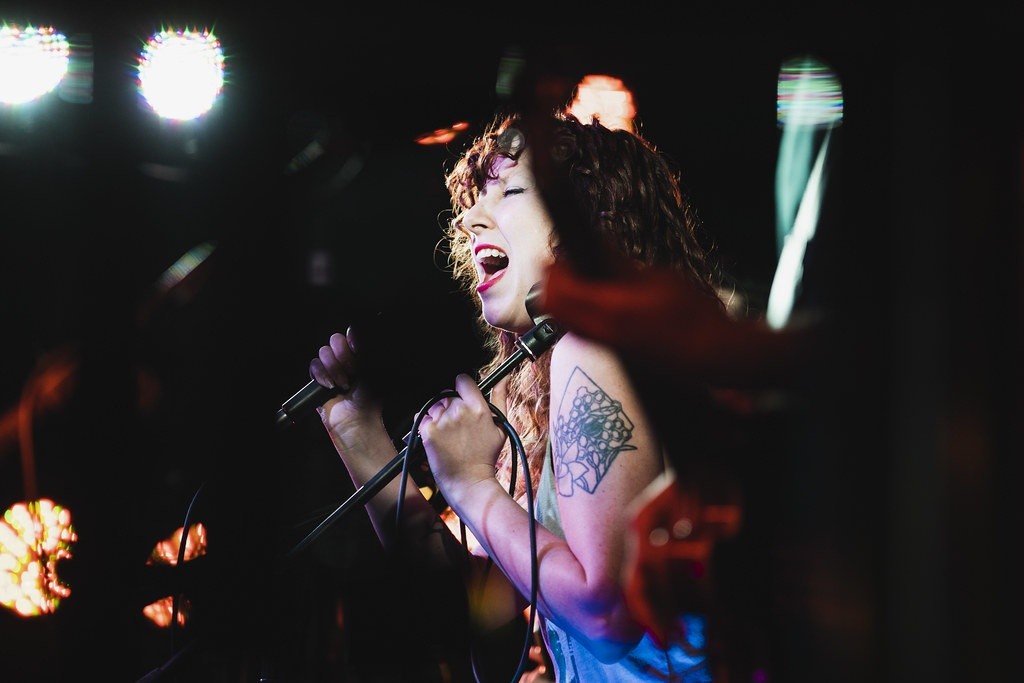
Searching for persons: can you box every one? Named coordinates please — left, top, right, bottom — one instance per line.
left=309, top=0, right=1024, bottom=683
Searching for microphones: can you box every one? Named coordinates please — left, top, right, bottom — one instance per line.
left=271, top=305, right=401, bottom=434
